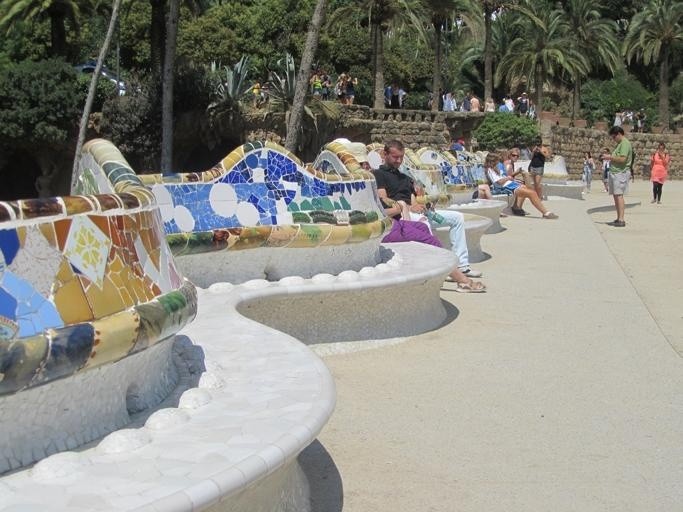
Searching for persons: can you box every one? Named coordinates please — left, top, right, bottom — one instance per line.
left=254, top=77, right=266, bottom=98
left=614, top=108, right=646, bottom=133
left=384, top=81, right=407, bottom=109
left=650, top=143, right=669, bottom=204
left=439, top=88, right=537, bottom=119
left=440, top=136, right=558, bottom=219
left=309, top=71, right=359, bottom=105
left=35, top=159, right=59, bottom=197
left=582, top=151, right=595, bottom=194
left=364, top=140, right=485, bottom=292
left=601, top=148, right=611, bottom=192
left=601, top=126, right=633, bottom=227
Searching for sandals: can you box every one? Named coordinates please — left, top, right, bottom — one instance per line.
left=456, top=279, right=486, bottom=292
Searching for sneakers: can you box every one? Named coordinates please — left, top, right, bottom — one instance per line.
left=512, top=207, right=524, bottom=216
left=608, top=220, right=625, bottom=226
left=543, top=211, right=557, bottom=218
left=458, top=266, right=481, bottom=277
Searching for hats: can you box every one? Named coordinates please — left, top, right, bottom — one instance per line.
left=521, top=92, right=529, bottom=97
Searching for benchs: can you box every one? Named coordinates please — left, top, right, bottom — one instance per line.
left=0, top=178, right=585, bottom=512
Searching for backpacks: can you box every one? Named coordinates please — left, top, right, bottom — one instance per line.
left=520, top=100, right=527, bottom=113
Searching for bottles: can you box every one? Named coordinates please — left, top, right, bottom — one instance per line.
left=426, top=211, right=445, bottom=225
left=581, top=191, right=585, bottom=200
